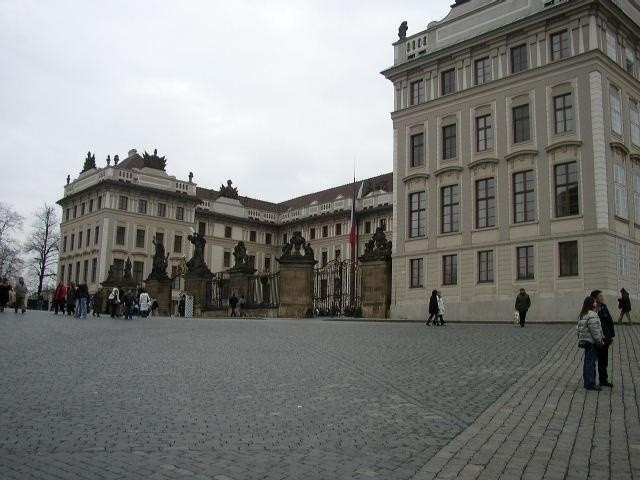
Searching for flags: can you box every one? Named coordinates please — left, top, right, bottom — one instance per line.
left=348, top=204, right=356, bottom=246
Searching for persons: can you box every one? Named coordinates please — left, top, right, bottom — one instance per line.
left=432, top=289, right=446, bottom=326
left=616, top=288, right=632, bottom=326
left=50, top=280, right=160, bottom=321
left=590, top=289, right=615, bottom=388
left=221, top=292, right=247, bottom=318
left=576, top=296, right=605, bottom=391
left=425, top=290, right=441, bottom=326
left=0, top=276, right=29, bottom=315
left=515, top=288, right=531, bottom=327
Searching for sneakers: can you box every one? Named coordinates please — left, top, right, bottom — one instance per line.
left=521, top=324, right=524, bottom=327
left=584, top=385, right=602, bottom=391
left=426, top=323, right=446, bottom=326
left=15, top=310, right=26, bottom=314
left=54, top=311, right=147, bottom=320
left=599, top=381, right=613, bottom=387
